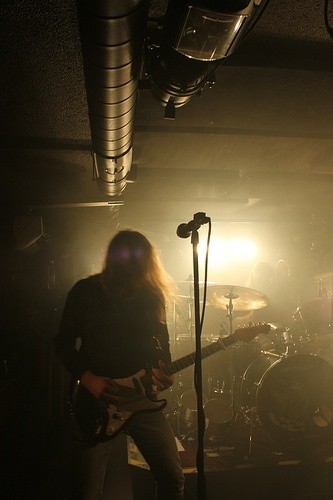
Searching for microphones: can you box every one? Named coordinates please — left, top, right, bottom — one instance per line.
left=177, top=217, right=210, bottom=238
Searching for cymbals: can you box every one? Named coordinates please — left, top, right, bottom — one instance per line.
left=195, top=283, right=271, bottom=309
left=171, top=280, right=207, bottom=299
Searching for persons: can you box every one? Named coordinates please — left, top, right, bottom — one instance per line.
left=252, top=260, right=296, bottom=306
left=55, top=229, right=186, bottom=500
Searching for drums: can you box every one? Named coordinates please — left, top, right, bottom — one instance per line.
left=169, top=332, right=207, bottom=363
left=209, top=333, right=265, bottom=396
left=240, top=349, right=333, bottom=440
left=200, top=373, right=234, bottom=423
left=179, top=368, right=209, bottom=410
left=288, top=299, right=333, bottom=346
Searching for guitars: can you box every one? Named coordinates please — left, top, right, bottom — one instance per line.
left=75, top=321, right=272, bottom=449
